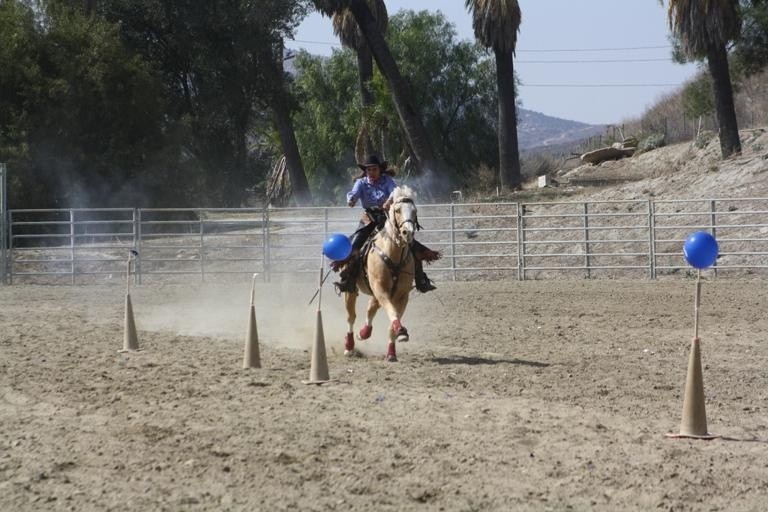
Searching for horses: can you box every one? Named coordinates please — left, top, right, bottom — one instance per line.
left=343, top=184, right=418, bottom=362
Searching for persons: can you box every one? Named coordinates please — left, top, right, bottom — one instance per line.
left=331, top=156, right=438, bottom=292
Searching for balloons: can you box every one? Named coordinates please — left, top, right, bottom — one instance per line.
left=682, top=231, right=719, bottom=270
left=323, top=233, right=352, bottom=262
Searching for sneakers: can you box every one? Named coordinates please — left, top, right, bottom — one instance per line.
left=333, top=279, right=355, bottom=293
left=417, top=284, right=436, bottom=293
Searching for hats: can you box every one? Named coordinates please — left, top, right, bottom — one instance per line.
left=356, top=155, right=388, bottom=175
